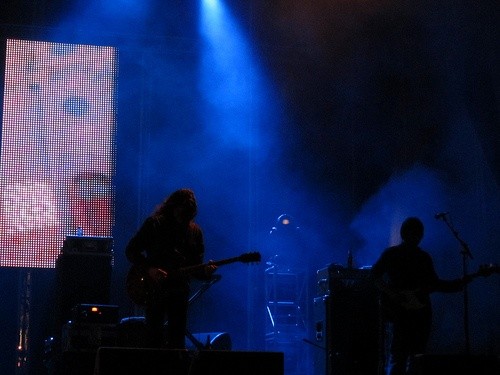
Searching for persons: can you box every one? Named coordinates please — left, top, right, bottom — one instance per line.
left=126, top=189, right=218, bottom=349
left=368, top=217, right=472, bottom=375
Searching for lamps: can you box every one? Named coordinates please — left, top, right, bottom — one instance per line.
left=270, top=213, right=305, bottom=262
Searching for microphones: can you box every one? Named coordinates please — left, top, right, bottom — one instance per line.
left=435, top=211, right=448, bottom=219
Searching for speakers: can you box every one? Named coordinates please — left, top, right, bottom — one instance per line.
left=312, top=295, right=384, bottom=375
left=63, top=235, right=112, bottom=323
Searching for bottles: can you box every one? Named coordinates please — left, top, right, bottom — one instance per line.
left=77, top=227, right=82, bottom=236
left=346, top=250, right=352, bottom=267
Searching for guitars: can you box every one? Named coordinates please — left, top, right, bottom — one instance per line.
left=126, top=251, right=261, bottom=299
left=382, top=264, right=500, bottom=323
